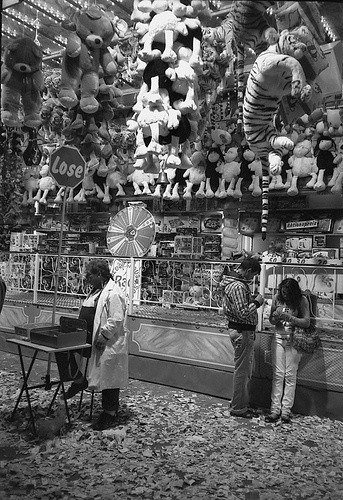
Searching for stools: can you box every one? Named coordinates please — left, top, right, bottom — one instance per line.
left=76, top=351, right=127, bottom=419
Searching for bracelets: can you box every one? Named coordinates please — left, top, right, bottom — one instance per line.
left=289, top=315, right=295, bottom=323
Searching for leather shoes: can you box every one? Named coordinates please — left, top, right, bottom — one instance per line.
left=94, top=411, right=119, bottom=430
left=61, top=377, right=88, bottom=399
left=230, top=409, right=260, bottom=418
left=247, top=402, right=257, bottom=408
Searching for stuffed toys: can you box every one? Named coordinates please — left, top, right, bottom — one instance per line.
left=0, top=0, right=343, bottom=208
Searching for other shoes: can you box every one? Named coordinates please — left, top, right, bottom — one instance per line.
left=280, top=413, right=290, bottom=424
left=265, top=412, right=279, bottom=424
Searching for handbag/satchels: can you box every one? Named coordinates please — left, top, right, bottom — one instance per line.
left=293, top=294, right=321, bottom=353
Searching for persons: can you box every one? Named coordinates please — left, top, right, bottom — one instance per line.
left=220, top=258, right=263, bottom=419
left=266, top=278, right=310, bottom=423
left=55, top=259, right=129, bottom=431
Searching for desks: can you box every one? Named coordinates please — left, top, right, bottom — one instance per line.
left=5, top=339, right=91, bottom=436
